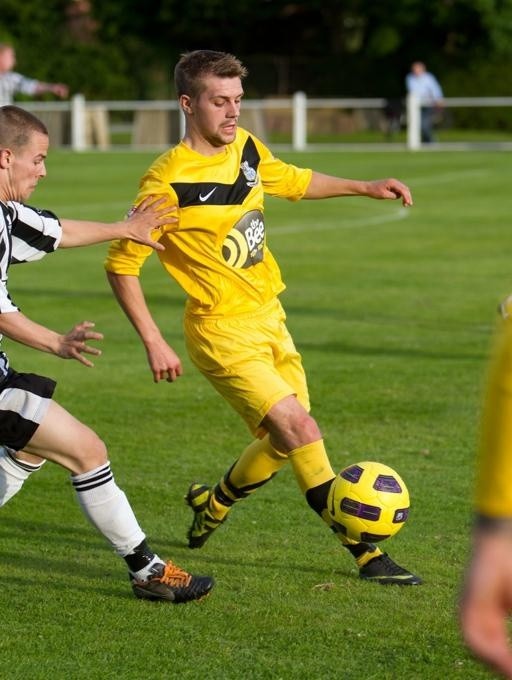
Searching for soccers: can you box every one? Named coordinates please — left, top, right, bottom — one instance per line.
left=326, top=460, right=409, bottom=543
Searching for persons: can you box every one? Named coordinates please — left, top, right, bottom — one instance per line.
left=0, top=103, right=215, bottom=603
left=0, top=40, right=69, bottom=107
left=104, top=48, right=426, bottom=587
left=458, top=293, right=512, bottom=679
left=406, top=60, right=445, bottom=141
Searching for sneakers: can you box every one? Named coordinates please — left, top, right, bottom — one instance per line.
left=127, top=553, right=216, bottom=604
left=183, top=482, right=228, bottom=549
left=358, top=553, right=422, bottom=585
